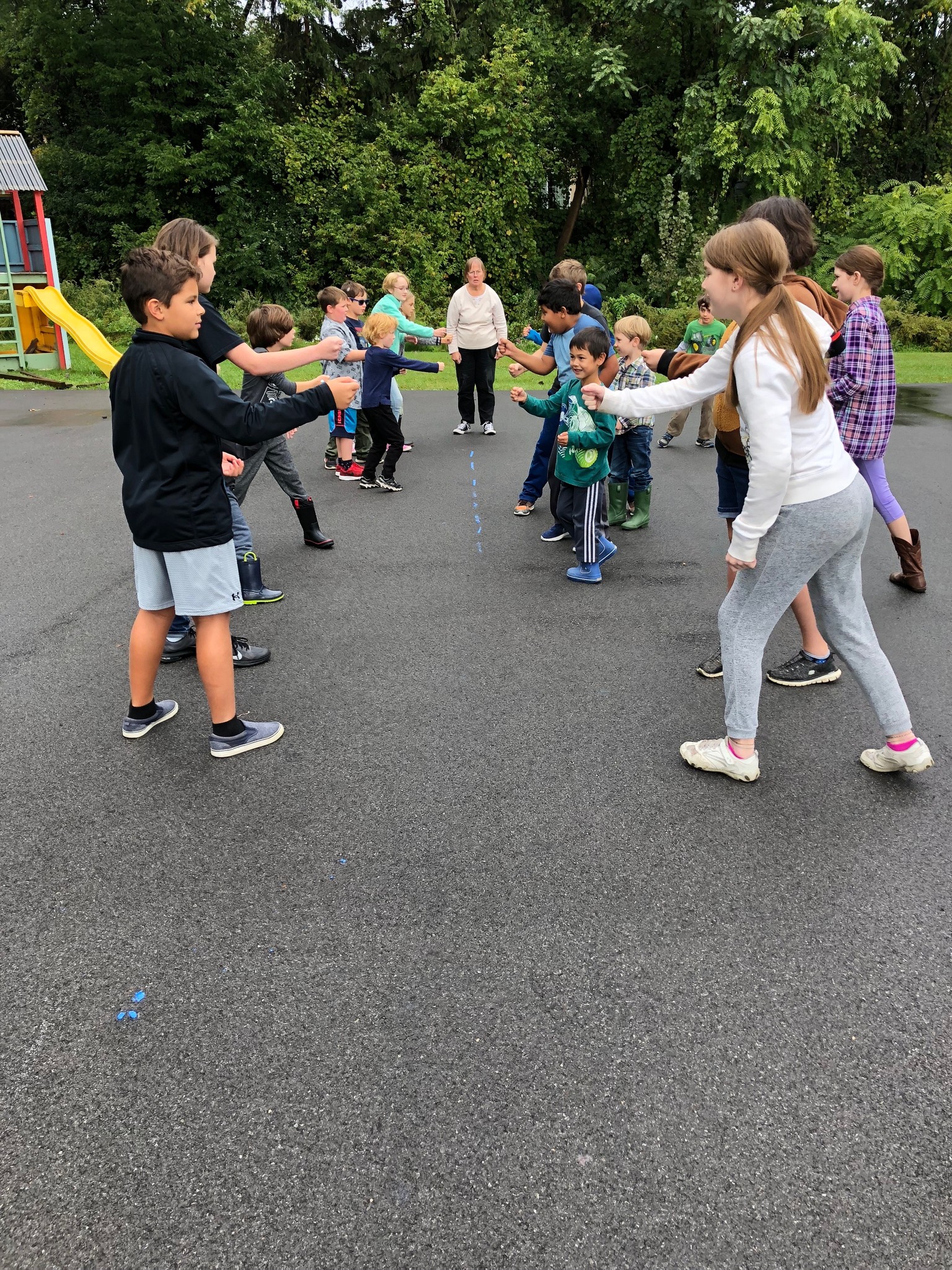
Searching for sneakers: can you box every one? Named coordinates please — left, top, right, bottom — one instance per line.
left=657, top=431, right=674, bottom=448
left=231, top=634, right=271, bottom=668
left=159, top=625, right=197, bottom=664
left=540, top=523, right=569, bottom=541
left=696, top=645, right=723, bottom=678
left=359, top=476, right=379, bottom=489
left=453, top=420, right=471, bottom=434
left=565, top=535, right=617, bottom=583
left=122, top=700, right=179, bottom=739
left=860, top=738, right=935, bottom=774
left=355, top=456, right=385, bottom=464
left=766, top=650, right=841, bottom=687
left=375, top=475, right=403, bottom=492
left=513, top=498, right=535, bottom=516
left=482, top=421, right=496, bottom=435
left=680, top=735, right=760, bottom=783
left=324, top=457, right=337, bottom=469
left=386, top=444, right=413, bottom=452
left=696, top=437, right=715, bottom=448
left=209, top=718, right=285, bottom=758
left=335, top=463, right=362, bottom=481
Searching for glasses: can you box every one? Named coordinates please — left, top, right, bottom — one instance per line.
left=349, top=296, right=370, bottom=306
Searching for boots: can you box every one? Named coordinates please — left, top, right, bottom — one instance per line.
left=292, top=497, right=335, bottom=548
left=889, top=527, right=927, bottom=593
left=237, top=552, right=285, bottom=606
left=602, top=481, right=652, bottom=530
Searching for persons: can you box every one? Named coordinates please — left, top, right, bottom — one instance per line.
left=821, top=245, right=927, bottom=592
left=639, top=197, right=841, bottom=689
left=578, top=217, right=935, bottom=783
left=658, top=295, right=727, bottom=448
left=227, top=302, right=335, bottom=549
left=109, top=217, right=362, bottom=759
left=447, top=256, right=507, bottom=436
left=317, top=272, right=454, bottom=490
left=498, top=258, right=658, bottom=584
